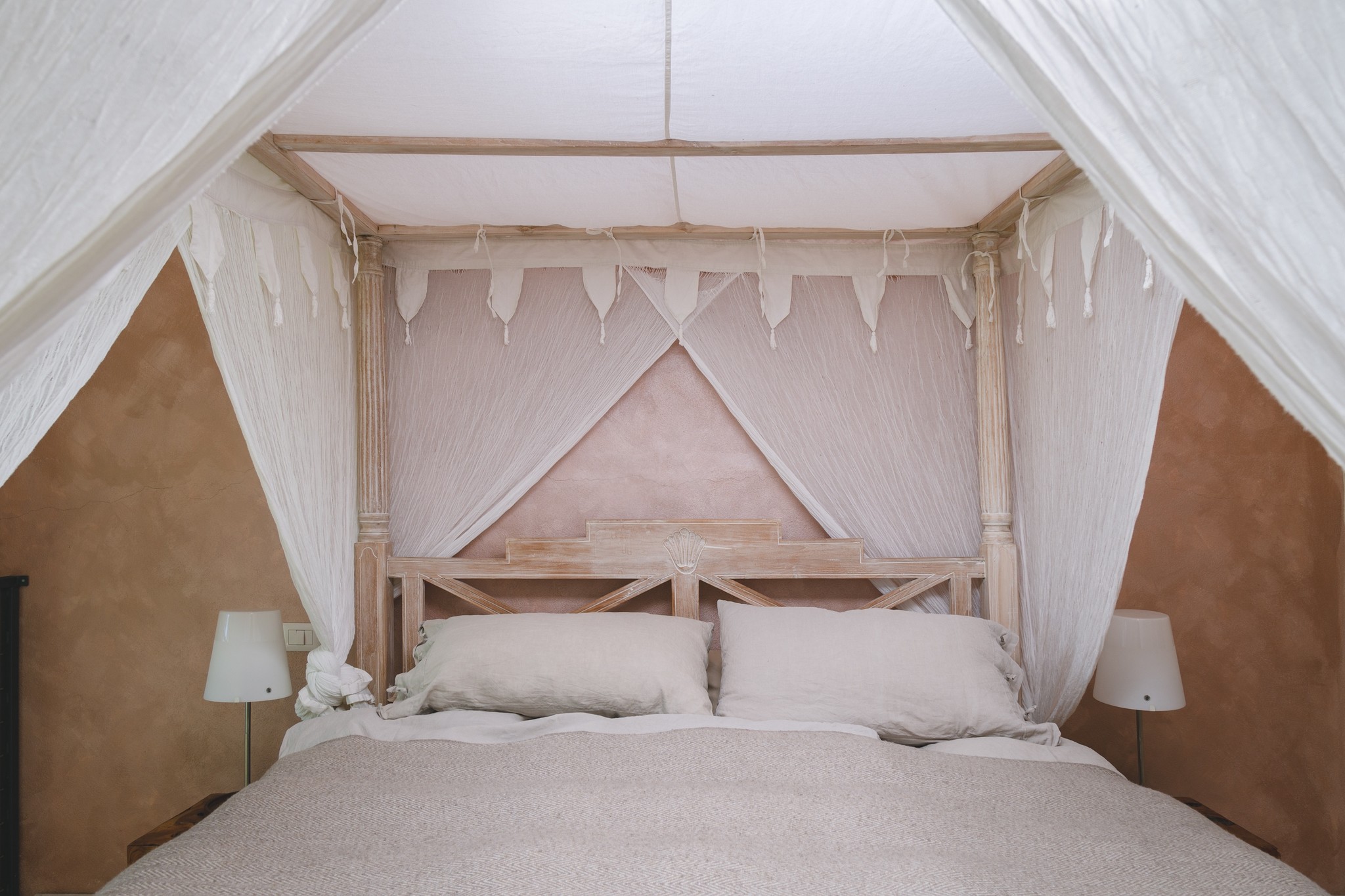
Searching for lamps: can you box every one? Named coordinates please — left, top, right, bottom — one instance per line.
left=1091, top=608, right=1188, bottom=786
left=201, top=610, right=294, bottom=786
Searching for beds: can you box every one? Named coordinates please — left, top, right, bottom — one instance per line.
left=84, top=515, right=1332, bottom=896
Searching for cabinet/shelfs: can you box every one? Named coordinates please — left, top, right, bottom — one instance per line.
left=0, top=574, right=30, bottom=896
left=125, top=792, right=229, bottom=869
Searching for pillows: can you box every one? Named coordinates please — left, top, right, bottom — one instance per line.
left=715, top=597, right=1064, bottom=747
left=374, top=611, right=715, bottom=719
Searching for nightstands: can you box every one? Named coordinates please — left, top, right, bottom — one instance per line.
left=1173, top=796, right=1281, bottom=857
